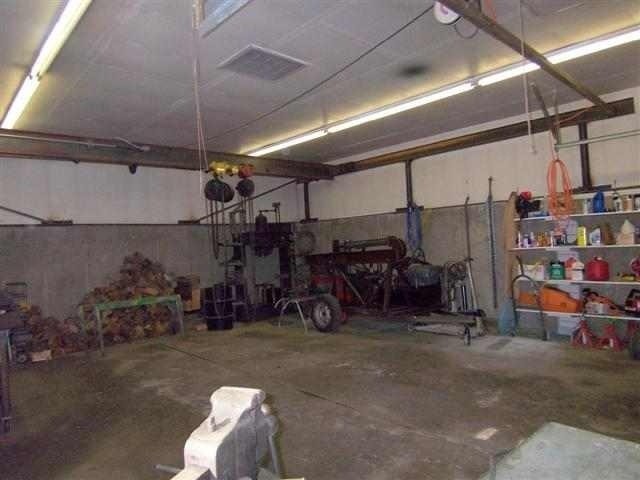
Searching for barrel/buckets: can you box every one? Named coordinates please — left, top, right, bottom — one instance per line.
left=204, top=286, right=235, bottom=331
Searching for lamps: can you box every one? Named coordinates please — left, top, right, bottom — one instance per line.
left=1, top=1, right=94, bottom=131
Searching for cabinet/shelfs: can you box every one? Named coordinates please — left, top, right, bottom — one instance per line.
left=497, top=191, right=640, bottom=336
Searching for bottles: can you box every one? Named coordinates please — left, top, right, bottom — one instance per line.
left=522, top=234, right=528, bottom=249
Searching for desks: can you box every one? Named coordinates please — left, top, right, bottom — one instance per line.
left=76, top=294, right=186, bottom=356
